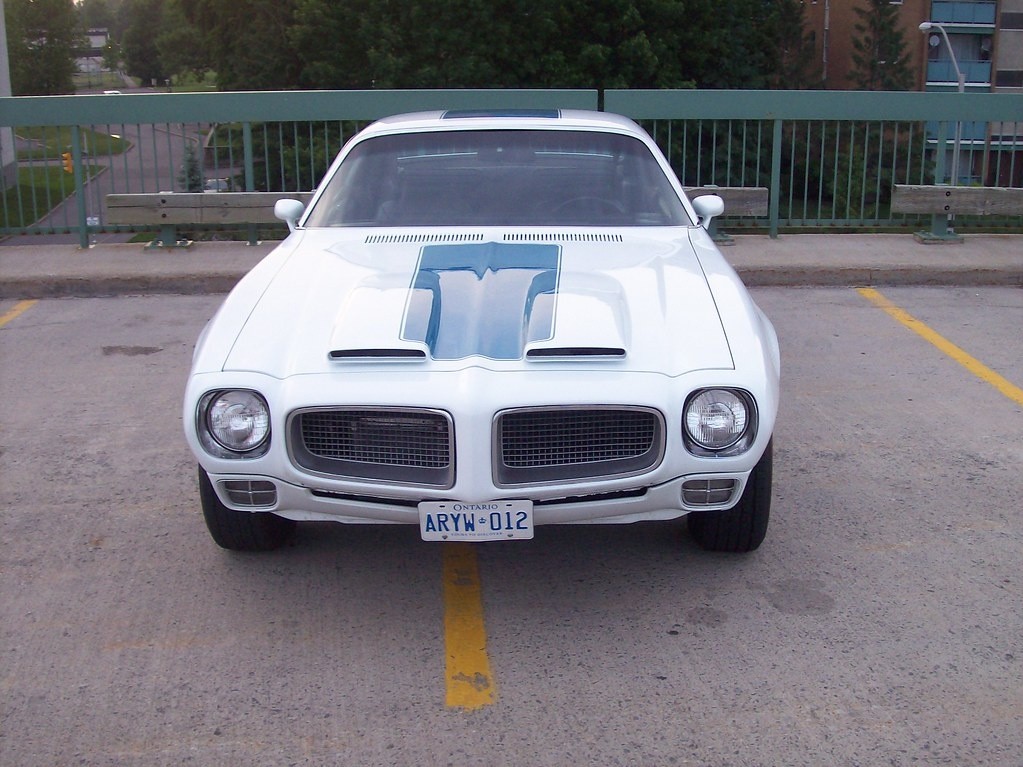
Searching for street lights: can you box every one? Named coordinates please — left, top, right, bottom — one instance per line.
left=920, top=21, right=965, bottom=228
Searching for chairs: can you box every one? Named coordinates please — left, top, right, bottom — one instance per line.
left=391, top=169, right=487, bottom=226
left=494, top=157, right=625, bottom=227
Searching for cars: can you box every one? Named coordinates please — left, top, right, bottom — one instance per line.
left=182, top=107, right=780, bottom=553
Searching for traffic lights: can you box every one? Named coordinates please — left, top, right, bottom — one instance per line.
left=63, top=151, right=72, bottom=173
